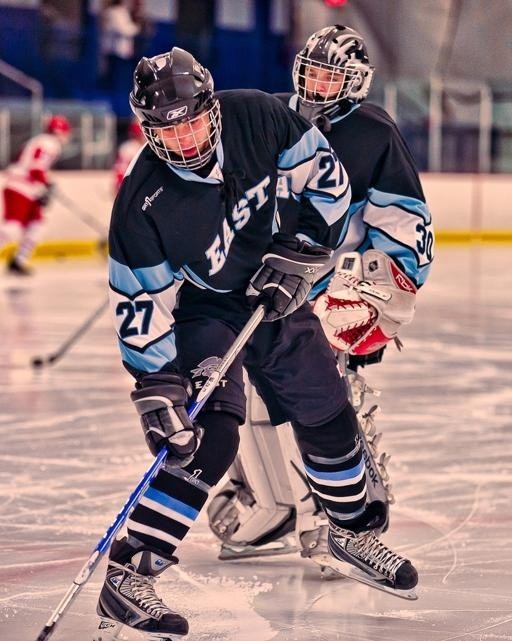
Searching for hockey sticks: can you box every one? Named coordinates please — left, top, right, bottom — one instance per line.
left=33, top=299, right=109, bottom=367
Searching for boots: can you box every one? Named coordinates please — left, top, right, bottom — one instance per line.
left=208, top=477, right=418, bottom=595
left=95, top=531, right=196, bottom=635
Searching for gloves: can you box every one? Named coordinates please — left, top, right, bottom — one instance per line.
left=129, top=366, right=202, bottom=470
left=245, top=232, right=332, bottom=323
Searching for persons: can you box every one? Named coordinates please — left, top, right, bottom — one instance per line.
left=96, top=0, right=143, bottom=122
left=1, top=115, right=72, bottom=278
left=95, top=45, right=419, bottom=638
left=113, top=117, right=149, bottom=196
left=205, top=24, right=436, bottom=560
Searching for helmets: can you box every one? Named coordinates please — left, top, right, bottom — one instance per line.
left=128, top=46, right=222, bottom=171
left=44, top=114, right=72, bottom=135
left=290, top=21, right=375, bottom=104
